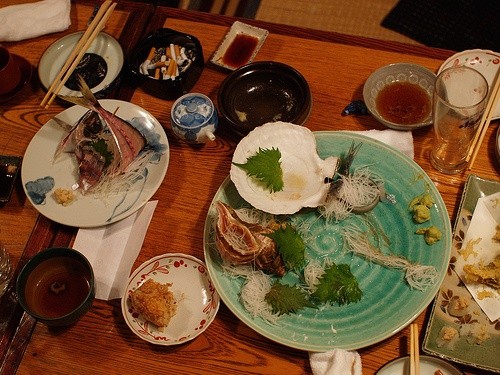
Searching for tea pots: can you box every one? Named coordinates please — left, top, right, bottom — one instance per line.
left=170, top=93, right=219, bottom=145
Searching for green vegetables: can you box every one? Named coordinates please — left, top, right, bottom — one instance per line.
left=232, top=146, right=284, bottom=193
left=91, top=136, right=115, bottom=169
left=261, top=222, right=363, bottom=315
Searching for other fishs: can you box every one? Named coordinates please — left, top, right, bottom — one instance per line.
left=52, top=75, right=150, bottom=194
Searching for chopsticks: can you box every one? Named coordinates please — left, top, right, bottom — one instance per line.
left=39, top=0, right=119, bottom=112
left=410, top=323, right=420, bottom=375
left=464, top=71, right=500, bottom=172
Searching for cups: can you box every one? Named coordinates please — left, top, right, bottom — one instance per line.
left=428, top=65, right=489, bottom=175
left=0, top=44, right=23, bottom=94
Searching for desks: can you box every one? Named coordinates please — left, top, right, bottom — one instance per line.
left=0, top=0, right=500, bottom=375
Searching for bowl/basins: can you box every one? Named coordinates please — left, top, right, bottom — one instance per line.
left=16, top=246, right=95, bottom=327
left=363, top=62, right=447, bottom=131
left=36, top=29, right=125, bottom=102
left=216, top=61, right=314, bottom=139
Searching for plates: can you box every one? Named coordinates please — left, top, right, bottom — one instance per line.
left=202, top=130, right=453, bottom=352
left=437, top=49, right=500, bottom=122
left=373, top=354, right=466, bottom=375
left=0, top=54, right=32, bottom=101
left=120, top=253, right=221, bottom=346
left=0, top=154, right=23, bottom=210
left=421, top=175, right=500, bottom=375
left=20, top=98, right=170, bottom=228
left=210, top=19, right=269, bottom=72
